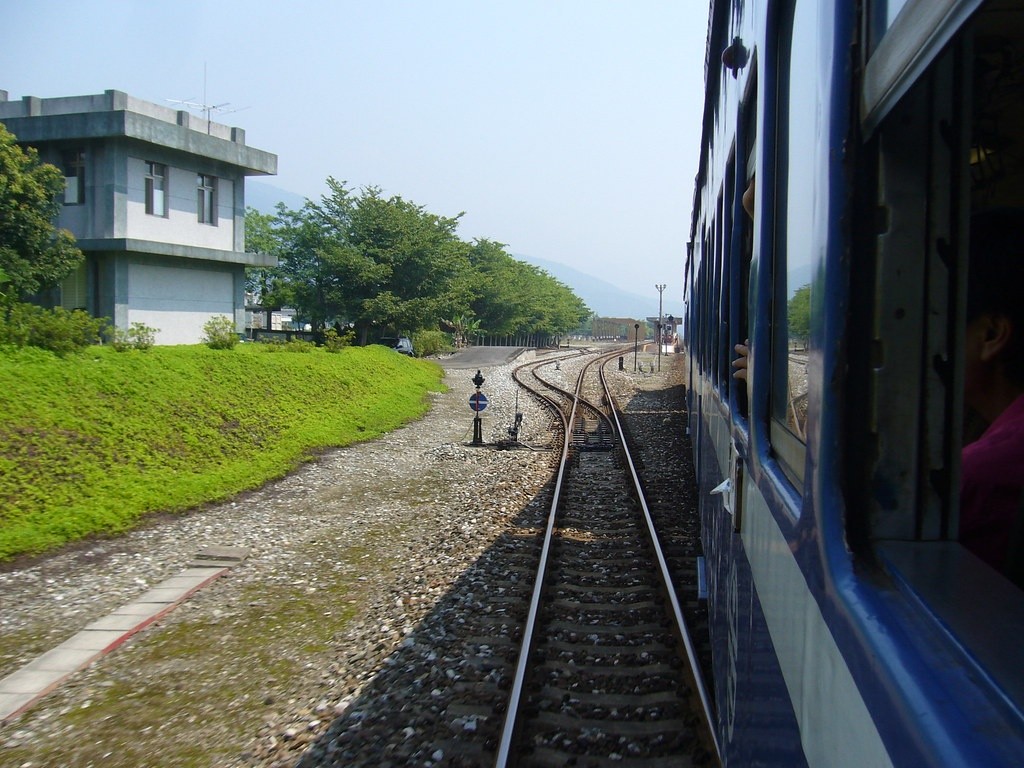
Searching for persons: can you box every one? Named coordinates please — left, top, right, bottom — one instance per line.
left=732, top=180, right=754, bottom=383
left=958, top=206, right=1024, bottom=574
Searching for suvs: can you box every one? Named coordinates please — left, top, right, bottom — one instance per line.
left=377, top=337, right=413, bottom=357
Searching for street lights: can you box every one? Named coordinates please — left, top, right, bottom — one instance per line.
left=654, top=284, right=667, bottom=353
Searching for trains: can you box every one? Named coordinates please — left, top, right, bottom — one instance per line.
left=685, top=0, right=1022, bottom=766
